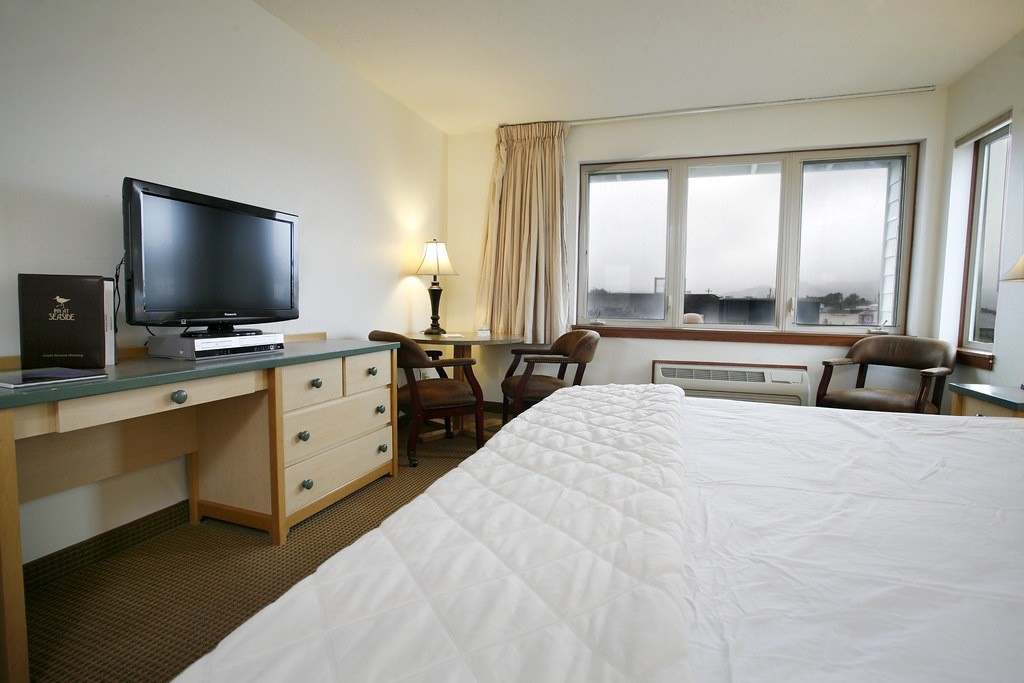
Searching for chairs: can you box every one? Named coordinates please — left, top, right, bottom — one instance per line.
left=501, top=329, right=601, bottom=427
left=368, top=329, right=485, bottom=469
left=815, top=335, right=957, bottom=416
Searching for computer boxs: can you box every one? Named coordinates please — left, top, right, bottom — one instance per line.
left=18, top=273, right=116, bottom=368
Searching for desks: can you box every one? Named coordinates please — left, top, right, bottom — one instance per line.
left=399, top=331, right=525, bottom=442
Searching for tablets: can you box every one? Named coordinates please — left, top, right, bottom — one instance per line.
left=0, top=366, right=108, bottom=389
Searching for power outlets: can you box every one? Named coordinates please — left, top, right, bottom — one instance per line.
left=420, top=369, right=430, bottom=381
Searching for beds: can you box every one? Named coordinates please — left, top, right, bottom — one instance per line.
left=165, top=382, right=1024, bottom=683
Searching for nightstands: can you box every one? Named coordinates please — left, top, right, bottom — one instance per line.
left=948, top=382, right=1024, bottom=419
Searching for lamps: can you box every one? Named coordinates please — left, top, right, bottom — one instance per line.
left=414, top=238, right=459, bottom=336
left=998, top=253, right=1024, bottom=389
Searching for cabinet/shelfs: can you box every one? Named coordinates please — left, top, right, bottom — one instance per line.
left=0, top=331, right=400, bottom=683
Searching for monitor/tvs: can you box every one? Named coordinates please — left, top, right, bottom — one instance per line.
left=122, top=176, right=300, bottom=326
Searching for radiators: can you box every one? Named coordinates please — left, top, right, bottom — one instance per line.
left=651, top=360, right=810, bottom=407
